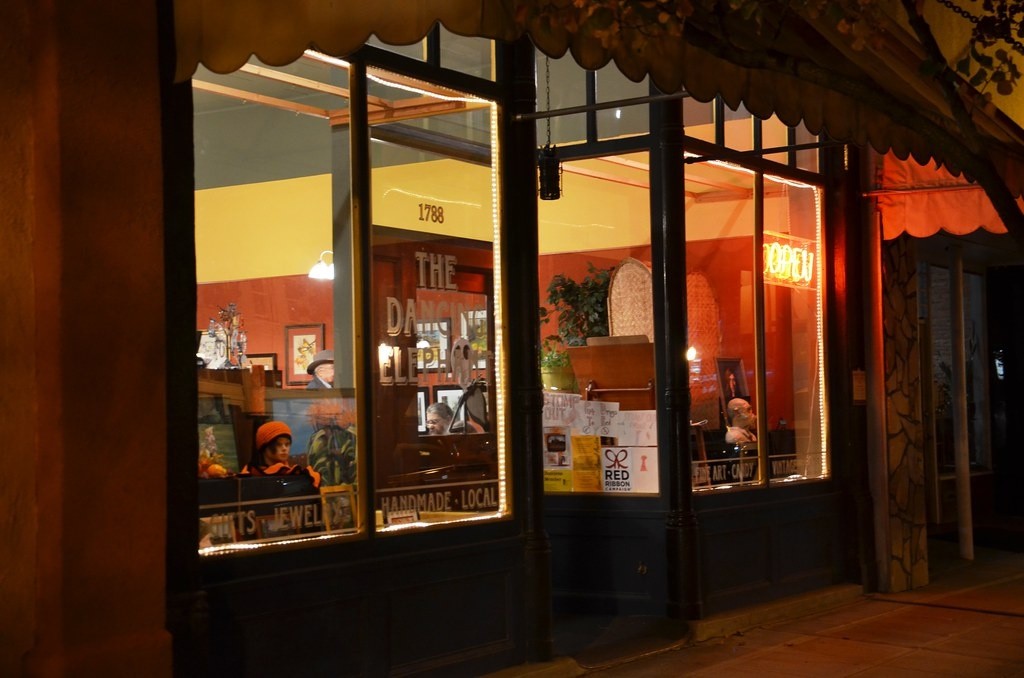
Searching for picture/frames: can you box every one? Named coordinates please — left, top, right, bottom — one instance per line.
left=714, top=357, right=752, bottom=430
left=285, top=323, right=326, bottom=386
left=417, top=387, right=430, bottom=434
left=239, top=353, right=277, bottom=371
left=320, top=484, right=357, bottom=535
left=415, top=317, right=452, bottom=373
left=263, top=388, right=358, bottom=530
left=459, top=308, right=487, bottom=370
left=433, top=385, right=489, bottom=433
left=197, top=329, right=231, bottom=368
left=382, top=505, right=421, bottom=524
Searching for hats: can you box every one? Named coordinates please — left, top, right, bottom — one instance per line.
left=256, top=422, right=291, bottom=451
left=307, top=350, right=334, bottom=375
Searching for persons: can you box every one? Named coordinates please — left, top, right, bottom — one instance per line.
left=724, top=367, right=737, bottom=399
left=425, top=402, right=476, bottom=434
left=307, top=351, right=335, bottom=390
left=725, top=399, right=757, bottom=443
left=240, top=421, right=322, bottom=490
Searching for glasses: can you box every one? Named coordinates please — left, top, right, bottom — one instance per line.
left=426, top=419, right=442, bottom=424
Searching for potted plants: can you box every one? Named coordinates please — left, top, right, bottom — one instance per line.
left=937, top=318, right=977, bottom=465
left=541, top=340, right=575, bottom=391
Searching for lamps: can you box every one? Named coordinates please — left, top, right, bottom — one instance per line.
left=308, top=251, right=336, bottom=280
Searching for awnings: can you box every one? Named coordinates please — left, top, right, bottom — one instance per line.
left=172, top=1, right=1024, bottom=244
